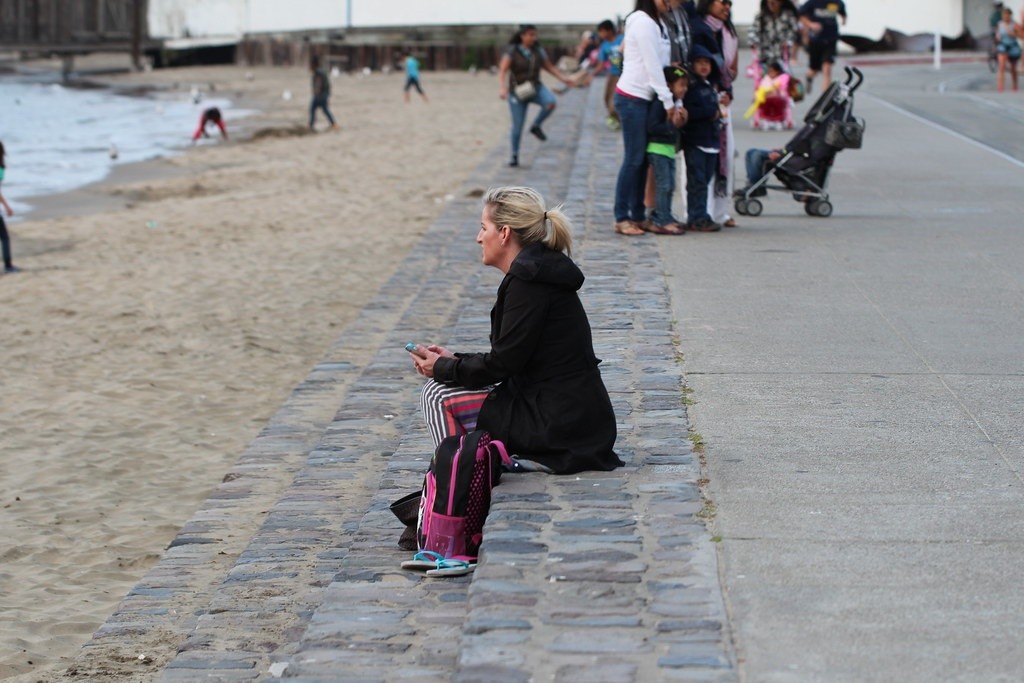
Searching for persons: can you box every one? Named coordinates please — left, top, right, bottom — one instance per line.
left=751, top=61, right=794, bottom=130
left=304, top=55, right=336, bottom=129
left=0, top=141, right=23, bottom=273
left=405, top=186, right=628, bottom=548
left=400, top=49, right=426, bottom=103
left=990, top=0, right=1024, bottom=92
left=613, top=0, right=739, bottom=235
left=191, top=98, right=229, bottom=143
left=747, top=0, right=846, bottom=93
left=495, top=24, right=577, bottom=167
left=553, top=19, right=625, bottom=131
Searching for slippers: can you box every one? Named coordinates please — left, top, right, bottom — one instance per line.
left=426, top=559, right=476, bottom=577
left=402, top=551, right=469, bottom=567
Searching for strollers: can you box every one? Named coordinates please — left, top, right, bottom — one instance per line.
left=732, top=65, right=866, bottom=217
left=753, top=43, right=792, bottom=130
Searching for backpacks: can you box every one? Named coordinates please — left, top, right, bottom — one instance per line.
left=417, top=430, right=512, bottom=564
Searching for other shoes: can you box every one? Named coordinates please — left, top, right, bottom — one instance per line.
left=650, top=223, right=685, bottom=234
left=672, top=219, right=686, bottom=229
left=510, top=155, right=518, bottom=165
left=725, top=218, right=736, bottom=228
left=633, top=220, right=651, bottom=232
left=530, top=126, right=545, bottom=141
left=614, top=222, right=645, bottom=235
left=687, top=218, right=721, bottom=231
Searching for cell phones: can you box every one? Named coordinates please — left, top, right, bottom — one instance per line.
left=404, top=343, right=427, bottom=360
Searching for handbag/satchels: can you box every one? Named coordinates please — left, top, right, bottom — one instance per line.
left=1009, top=45, right=1022, bottom=60
left=825, top=97, right=864, bottom=149
left=514, top=80, right=536, bottom=101
left=789, top=75, right=804, bottom=103
